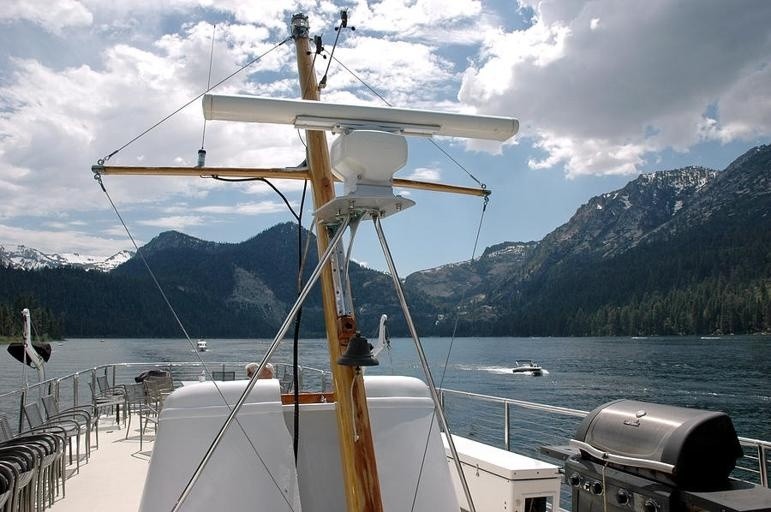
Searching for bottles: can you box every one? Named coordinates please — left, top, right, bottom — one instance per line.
left=200, top=369, right=206, bottom=383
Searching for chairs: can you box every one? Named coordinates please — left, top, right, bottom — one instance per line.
left=0, top=394, right=100, bottom=512
left=88, top=371, right=183, bottom=451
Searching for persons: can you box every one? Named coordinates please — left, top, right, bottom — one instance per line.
left=258, top=362, right=275, bottom=379
left=245, top=361, right=260, bottom=380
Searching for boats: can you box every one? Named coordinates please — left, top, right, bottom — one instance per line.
left=513, top=359, right=543, bottom=377
left=2, top=5, right=771, bottom=512
left=196, top=336, right=208, bottom=352
